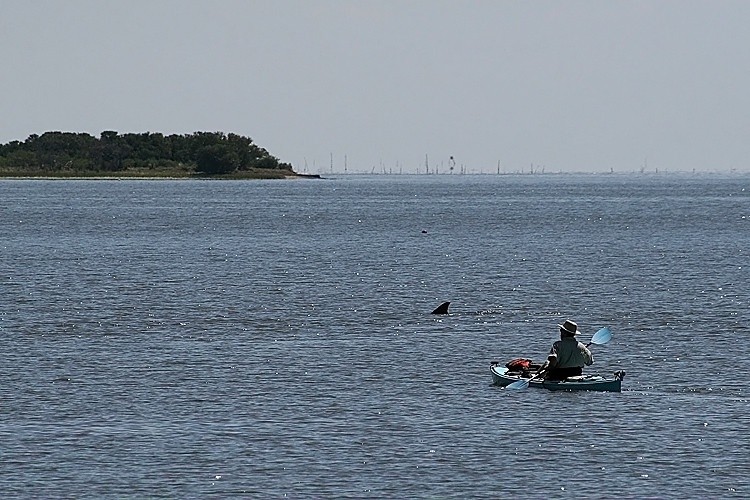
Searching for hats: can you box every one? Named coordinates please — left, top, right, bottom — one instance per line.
left=557, top=320, right=581, bottom=335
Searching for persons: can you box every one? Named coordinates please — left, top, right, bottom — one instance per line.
left=539, top=320, right=595, bottom=380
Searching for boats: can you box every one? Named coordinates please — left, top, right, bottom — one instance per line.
left=490, top=360, right=626, bottom=393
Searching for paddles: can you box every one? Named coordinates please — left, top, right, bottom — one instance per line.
left=505, top=327, right=612, bottom=390
